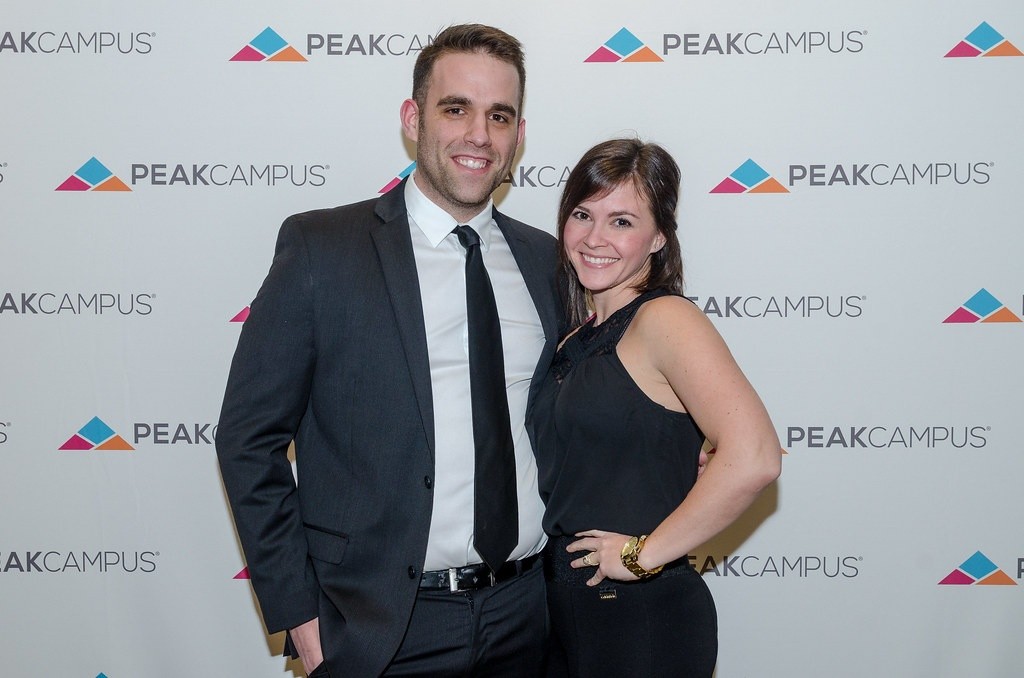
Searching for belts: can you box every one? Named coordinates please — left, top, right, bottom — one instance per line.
left=417, top=555, right=536, bottom=593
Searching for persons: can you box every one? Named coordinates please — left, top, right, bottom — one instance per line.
left=524, top=138, right=781, bottom=678
left=214, top=22, right=709, bottom=678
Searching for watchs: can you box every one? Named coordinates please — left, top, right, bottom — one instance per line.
left=620, top=534, right=664, bottom=578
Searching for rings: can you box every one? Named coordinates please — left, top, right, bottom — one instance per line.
left=583, top=552, right=599, bottom=566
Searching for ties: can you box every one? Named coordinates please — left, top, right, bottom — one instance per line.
left=453, top=225, right=521, bottom=573
